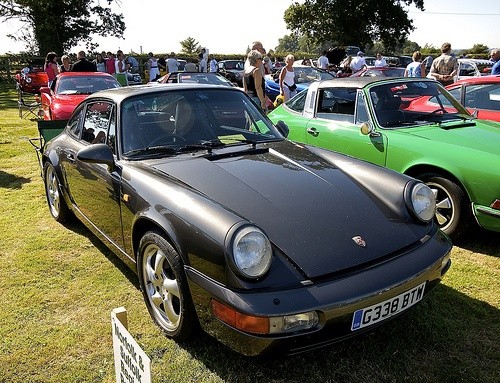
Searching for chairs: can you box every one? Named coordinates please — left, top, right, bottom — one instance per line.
left=374, top=97, right=405, bottom=123
left=175, top=100, right=207, bottom=140
left=17, top=89, right=42, bottom=118
left=334, top=101, right=355, bottom=115
left=474, top=90, right=491, bottom=110
left=29, top=120, right=67, bottom=164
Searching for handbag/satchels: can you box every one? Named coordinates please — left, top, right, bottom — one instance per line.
left=284, top=82, right=296, bottom=92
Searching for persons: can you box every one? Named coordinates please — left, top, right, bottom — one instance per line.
left=198, top=48, right=208, bottom=64
left=316, top=50, right=330, bottom=73
left=209, top=54, right=219, bottom=73
left=184, top=58, right=198, bottom=73
left=350, top=51, right=367, bottom=75
left=147, top=52, right=160, bottom=82
left=491, top=48, right=500, bottom=75
left=155, top=52, right=180, bottom=75
left=238, top=59, right=243, bottom=69
left=21, top=63, right=33, bottom=74
left=374, top=52, right=388, bottom=67
left=430, top=43, right=458, bottom=91
left=279, top=54, right=297, bottom=102
left=422, top=55, right=434, bottom=70
left=242, top=41, right=282, bottom=112
left=44, top=50, right=139, bottom=89
left=198, top=54, right=207, bottom=73
left=227, top=63, right=233, bottom=68
left=404, top=51, right=427, bottom=88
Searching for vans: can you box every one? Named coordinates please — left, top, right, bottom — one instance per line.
left=144, top=59, right=187, bottom=80
left=425, top=59, right=495, bottom=83
left=454, top=58, right=498, bottom=82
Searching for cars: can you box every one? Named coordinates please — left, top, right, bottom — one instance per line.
left=249, top=76, right=500, bottom=238
left=402, top=75, right=500, bottom=122
left=293, top=59, right=336, bottom=78
left=93, top=58, right=141, bottom=82
left=36, top=72, right=145, bottom=125
left=351, top=66, right=437, bottom=109
left=328, top=46, right=360, bottom=58
left=218, top=60, right=246, bottom=87
left=15, top=64, right=58, bottom=94
left=147, top=71, right=261, bottom=130
left=264, top=65, right=338, bottom=110
left=363, top=55, right=413, bottom=69
left=41, top=84, right=453, bottom=356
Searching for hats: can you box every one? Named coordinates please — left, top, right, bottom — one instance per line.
left=358, top=51, right=364, bottom=55
